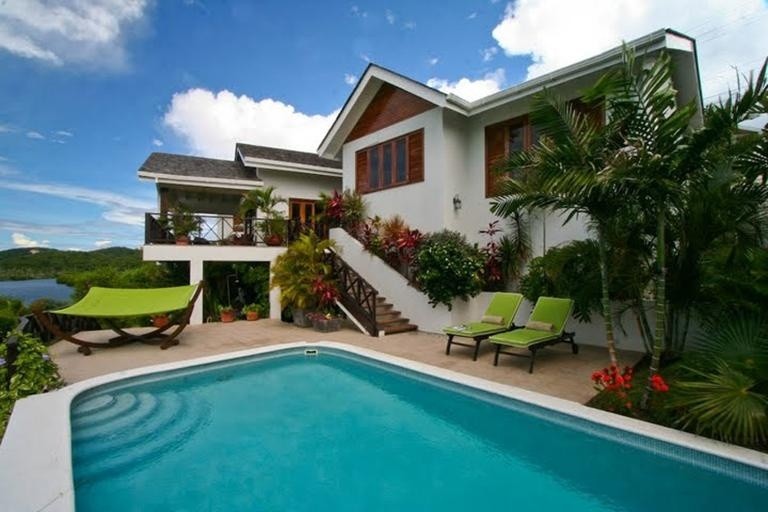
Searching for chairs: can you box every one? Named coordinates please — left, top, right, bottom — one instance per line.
left=442, top=291, right=578, bottom=373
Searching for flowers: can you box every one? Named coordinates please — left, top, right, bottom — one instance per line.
left=591, top=364, right=671, bottom=416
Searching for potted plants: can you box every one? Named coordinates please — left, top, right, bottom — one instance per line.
left=155, top=201, right=205, bottom=245
left=254, top=211, right=288, bottom=246
left=217, top=303, right=262, bottom=322
left=265, top=228, right=345, bottom=332
